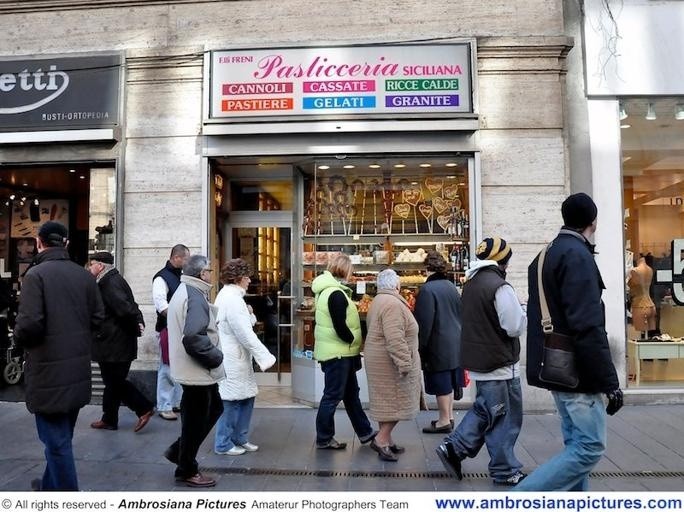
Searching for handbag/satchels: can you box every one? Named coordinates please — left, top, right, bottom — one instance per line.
left=538, top=332, right=587, bottom=389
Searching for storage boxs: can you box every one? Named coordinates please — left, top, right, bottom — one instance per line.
left=97, top=233, right=114, bottom=252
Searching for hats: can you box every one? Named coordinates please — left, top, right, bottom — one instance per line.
left=475, top=237, right=512, bottom=265
left=38, top=221, right=67, bottom=243
left=90, top=252, right=114, bottom=264
left=562, top=193, right=597, bottom=229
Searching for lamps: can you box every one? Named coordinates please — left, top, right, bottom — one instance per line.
left=619, top=101, right=629, bottom=121
left=645, top=102, right=657, bottom=120
left=674, top=99, right=684, bottom=122
left=620, top=112, right=632, bottom=129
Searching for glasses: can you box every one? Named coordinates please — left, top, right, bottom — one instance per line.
left=204, top=269, right=214, bottom=274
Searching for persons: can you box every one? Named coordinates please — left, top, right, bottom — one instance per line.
left=627, top=256, right=657, bottom=332
left=507, top=193, right=624, bottom=491
left=12, top=220, right=106, bottom=492
left=152, top=243, right=190, bottom=420
left=310, top=255, right=380, bottom=450
left=213, top=258, right=277, bottom=455
left=363, top=268, right=429, bottom=463
left=413, top=251, right=470, bottom=434
left=434, top=236, right=528, bottom=486
left=162, top=254, right=223, bottom=487
left=88, top=252, right=157, bottom=432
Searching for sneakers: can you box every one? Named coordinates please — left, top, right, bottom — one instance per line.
left=435, top=441, right=462, bottom=481
left=214, top=445, right=247, bottom=456
left=30, top=478, right=43, bottom=491
left=159, top=407, right=178, bottom=420
left=493, top=470, right=527, bottom=487
left=240, top=442, right=259, bottom=451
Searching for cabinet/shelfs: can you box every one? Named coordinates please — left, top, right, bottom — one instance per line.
left=626, top=338, right=684, bottom=388
left=301, top=232, right=470, bottom=353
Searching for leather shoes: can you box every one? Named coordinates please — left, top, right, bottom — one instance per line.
left=316, top=430, right=405, bottom=463
left=431, top=419, right=454, bottom=429
left=423, top=422, right=452, bottom=433
left=175, top=471, right=216, bottom=487
left=135, top=409, right=155, bottom=432
left=91, top=420, right=115, bottom=430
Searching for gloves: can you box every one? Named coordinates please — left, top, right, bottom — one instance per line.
left=606, top=387, right=623, bottom=416
